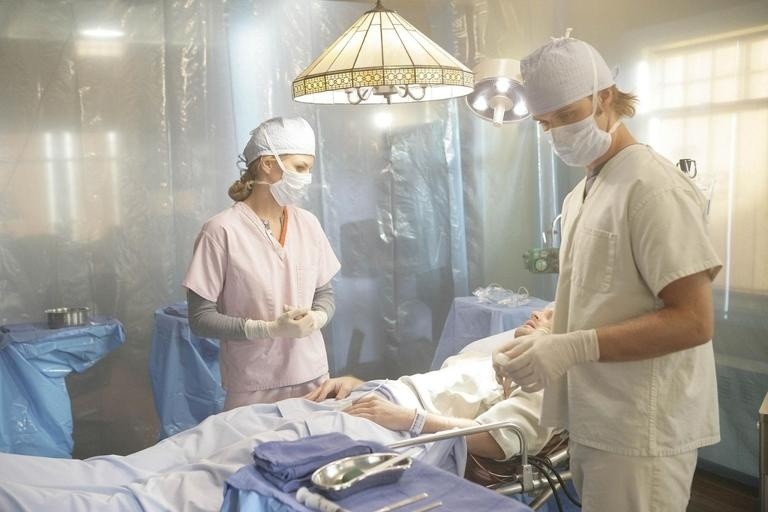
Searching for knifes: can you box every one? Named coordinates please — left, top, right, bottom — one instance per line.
left=373, top=489, right=428, bottom=511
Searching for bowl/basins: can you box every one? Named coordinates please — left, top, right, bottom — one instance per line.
left=43, top=305, right=91, bottom=329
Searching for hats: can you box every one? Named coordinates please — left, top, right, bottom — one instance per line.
left=242, top=115, right=315, bottom=167
left=519, top=38, right=616, bottom=118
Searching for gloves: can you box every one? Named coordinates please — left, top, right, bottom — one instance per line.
left=491, top=328, right=550, bottom=385
left=283, top=304, right=327, bottom=332
left=502, top=329, right=600, bottom=394
left=244, top=308, right=315, bottom=342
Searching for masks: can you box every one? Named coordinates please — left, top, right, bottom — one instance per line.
left=259, top=157, right=312, bottom=208
left=543, top=89, right=619, bottom=168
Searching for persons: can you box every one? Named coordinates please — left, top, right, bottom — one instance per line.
left=184, top=112, right=344, bottom=406
left=503, top=34, right=718, bottom=511
left=2, top=302, right=562, bottom=510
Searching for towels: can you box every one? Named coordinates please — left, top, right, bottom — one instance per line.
left=252, top=432, right=373, bottom=494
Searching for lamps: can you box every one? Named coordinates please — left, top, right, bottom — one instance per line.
left=292, top=0, right=474, bottom=106
left=465, top=59, right=555, bottom=251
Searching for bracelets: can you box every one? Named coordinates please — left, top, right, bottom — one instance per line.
left=408, top=405, right=427, bottom=435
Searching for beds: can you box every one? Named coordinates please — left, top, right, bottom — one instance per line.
left=387, top=422, right=572, bottom=512
